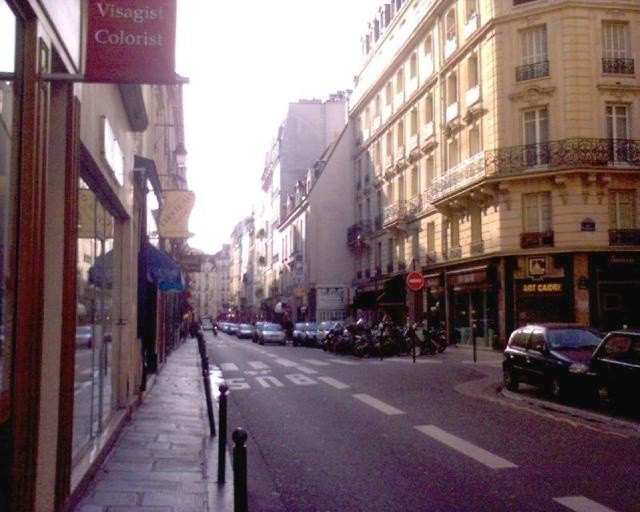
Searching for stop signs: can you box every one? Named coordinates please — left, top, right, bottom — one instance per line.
left=406, top=271, right=424, bottom=292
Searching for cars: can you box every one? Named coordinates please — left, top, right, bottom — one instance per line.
left=502, top=322, right=616, bottom=399
left=203, top=322, right=214, bottom=331
left=290, top=322, right=305, bottom=345
left=235, top=323, right=255, bottom=338
left=258, top=323, right=288, bottom=346
left=215, top=321, right=238, bottom=335
left=590, top=329, right=640, bottom=408
left=300, top=322, right=318, bottom=346
left=315, top=320, right=338, bottom=344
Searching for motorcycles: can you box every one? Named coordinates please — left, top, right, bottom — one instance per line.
left=320, top=321, right=451, bottom=360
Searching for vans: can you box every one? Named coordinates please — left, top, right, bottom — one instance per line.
left=252, top=321, right=268, bottom=343
